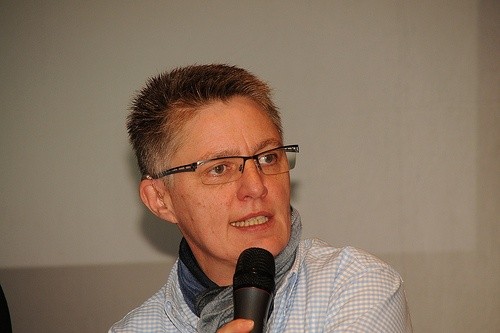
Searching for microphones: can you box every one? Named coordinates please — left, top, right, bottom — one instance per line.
left=233, top=247, right=275, bottom=333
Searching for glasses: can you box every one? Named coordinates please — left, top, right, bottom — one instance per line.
left=141, top=145, right=299, bottom=185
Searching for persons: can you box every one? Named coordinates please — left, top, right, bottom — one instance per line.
left=106, top=64, right=413, bottom=333
